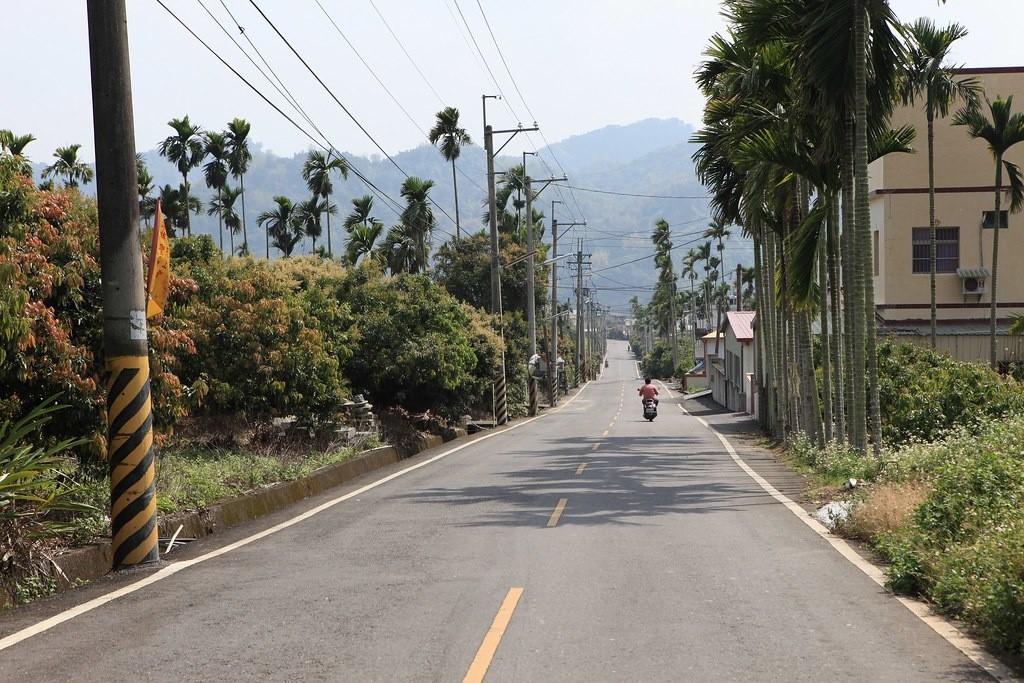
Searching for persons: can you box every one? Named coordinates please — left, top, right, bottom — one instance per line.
left=639, top=378, right=659, bottom=406
left=605, top=359, right=608, bottom=365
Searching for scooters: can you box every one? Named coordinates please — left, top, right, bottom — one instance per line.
left=638, top=387, right=660, bottom=422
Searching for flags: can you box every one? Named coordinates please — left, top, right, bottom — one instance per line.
left=146, top=206, right=170, bottom=319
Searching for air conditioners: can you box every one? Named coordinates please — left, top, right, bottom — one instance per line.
left=963, top=278, right=986, bottom=295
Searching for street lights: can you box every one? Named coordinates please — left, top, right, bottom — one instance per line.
left=527, top=254, right=574, bottom=417
left=491, top=249, right=539, bottom=425
left=542, top=310, right=569, bottom=406
